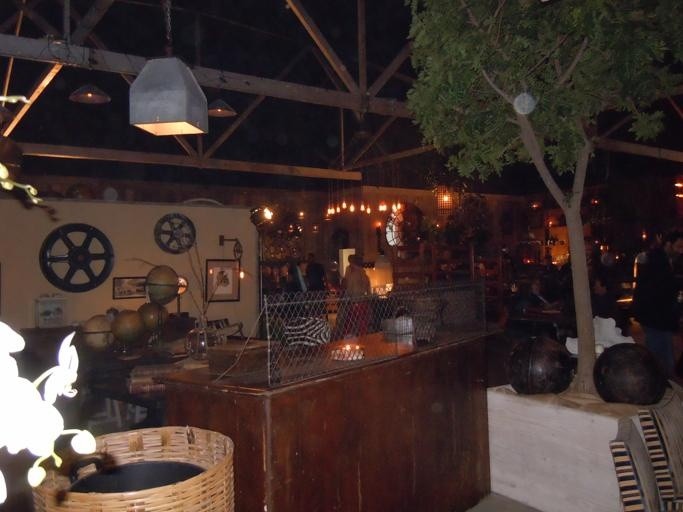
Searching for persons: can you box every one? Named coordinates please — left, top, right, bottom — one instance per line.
left=528, top=281, right=566, bottom=307
left=304, top=251, right=329, bottom=321
left=339, top=255, right=371, bottom=340
left=344, top=256, right=350, bottom=283
left=632, top=230, right=682, bottom=380
left=541, top=254, right=557, bottom=273
left=553, top=235, right=623, bottom=339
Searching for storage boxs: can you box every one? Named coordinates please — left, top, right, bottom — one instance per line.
left=208, top=340, right=279, bottom=378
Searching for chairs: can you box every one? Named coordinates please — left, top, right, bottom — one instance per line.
left=637, top=391, right=683, bottom=510
left=273, top=316, right=331, bottom=351
left=609, top=418, right=683, bottom=512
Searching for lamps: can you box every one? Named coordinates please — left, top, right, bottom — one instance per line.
left=129, top=0, right=209, bottom=137
left=249, top=202, right=280, bottom=234
left=68, top=83, right=111, bottom=104
left=209, top=99, right=238, bottom=117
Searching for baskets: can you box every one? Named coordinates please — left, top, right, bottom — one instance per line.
left=29, top=425, right=235, bottom=511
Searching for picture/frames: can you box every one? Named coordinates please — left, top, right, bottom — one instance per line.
left=113, top=276, right=147, bottom=299
left=205, top=259, right=240, bottom=302
